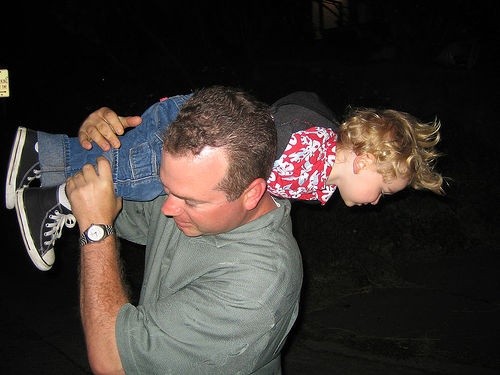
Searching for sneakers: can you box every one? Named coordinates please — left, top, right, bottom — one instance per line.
left=5, top=126, right=41, bottom=209
left=15, top=183, right=77, bottom=272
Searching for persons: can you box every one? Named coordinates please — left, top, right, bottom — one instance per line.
left=66, top=85, right=304, bottom=375
left=6, top=91, right=455, bottom=271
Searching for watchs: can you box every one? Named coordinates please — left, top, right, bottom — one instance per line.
left=79, top=222, right=116, bottom=247
